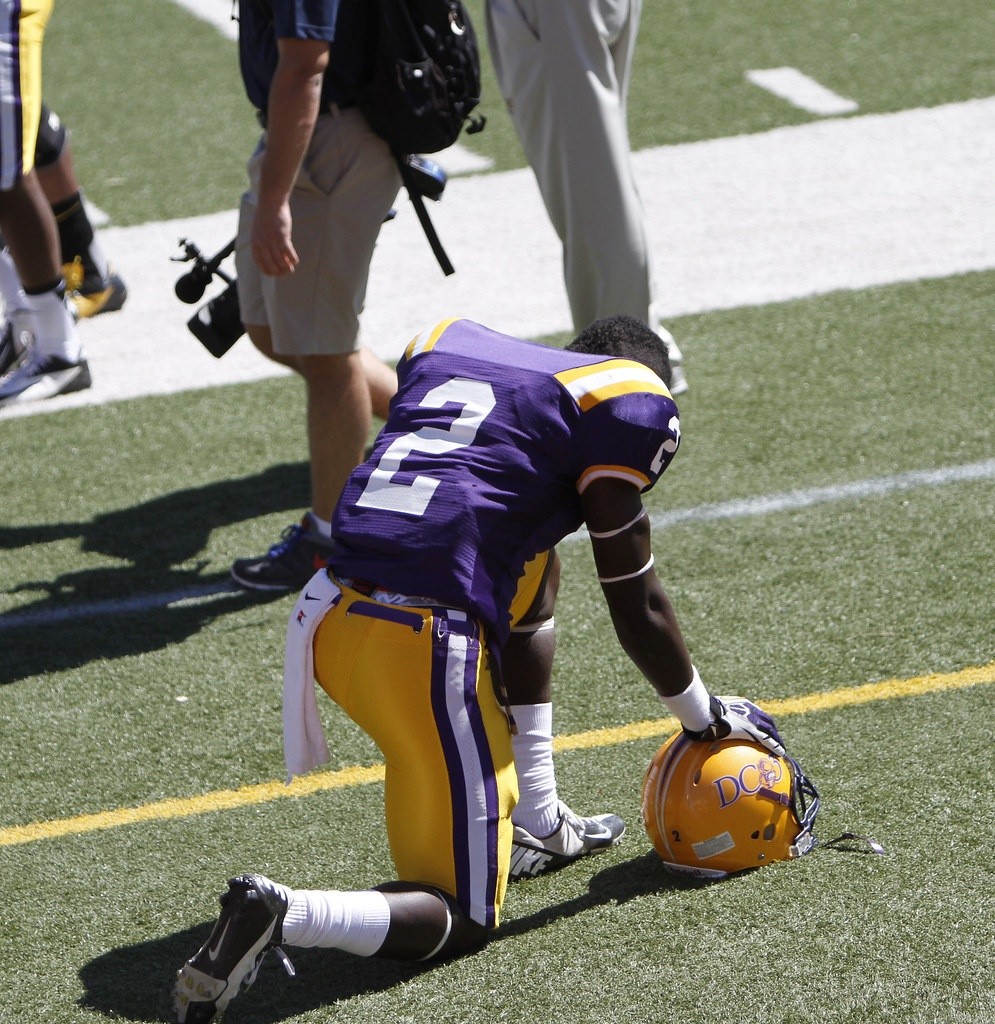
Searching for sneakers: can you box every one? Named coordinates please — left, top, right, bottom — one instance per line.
left=170, top=873, right=298, bottom=1024
left=509, top=800, right=626, bottom=879
left=0, top=351, right=91, bottom=408
left=71, top=275, right=127, bottom=319
left=231, top=514, right=335, bottom=590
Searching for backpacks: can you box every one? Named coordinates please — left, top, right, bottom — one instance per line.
left=359, top=0, right=484, bottom=156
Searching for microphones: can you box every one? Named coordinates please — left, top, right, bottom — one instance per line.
left=175, top=264, right=211, bottom=304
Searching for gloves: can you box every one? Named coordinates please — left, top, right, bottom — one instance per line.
left=681, top=694, right=786, bottom=756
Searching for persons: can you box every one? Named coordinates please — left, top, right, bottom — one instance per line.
left=0, top=0, right=92, bottom=406
left=230, top=0, right=408, bottom=593
left=483, top=0, right=689, bottom=396
left=34, top=104, right=126, bottom=322
left=173, top=316, right=785, bottom=1024
